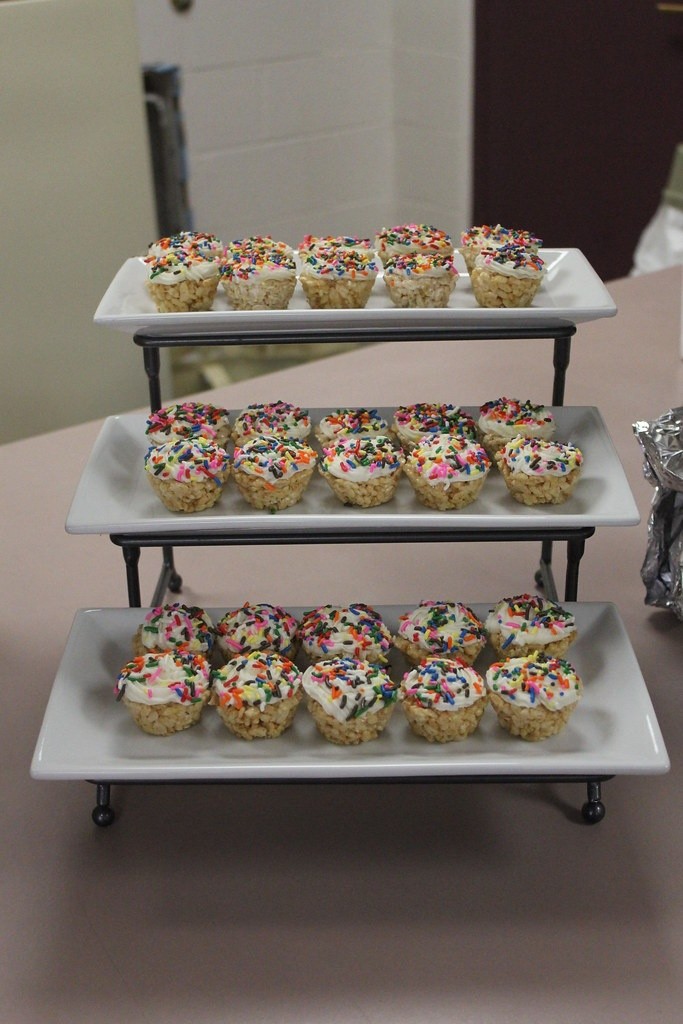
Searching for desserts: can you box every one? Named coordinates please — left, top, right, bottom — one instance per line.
left=143, top=224, right=548, bottom=310
left=144, top=397, right=583, bottom=504
left=114, top=594, right=582, bottom=743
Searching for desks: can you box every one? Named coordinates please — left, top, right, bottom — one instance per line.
left=0, top=261, right=683, bottom=1024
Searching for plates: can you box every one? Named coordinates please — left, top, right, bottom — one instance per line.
left=93, top=247, right=615, bottom=319
left=65, top=408, right=641, bottom=525
left=24, top=594, right=672, bottom=777
